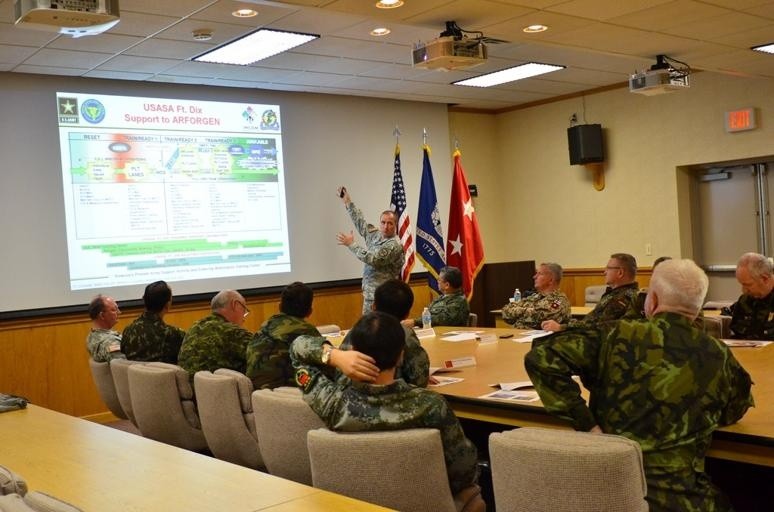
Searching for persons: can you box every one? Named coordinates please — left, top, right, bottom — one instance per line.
left=336, top=185, right=406, bottom=315
left=177, top=289, right=253, bottom=382
left=84, top=294, right=126, bottom=363
left=120, top=281, right=186, bottom=366
left=244, top=282, right=321, bottom=390
left=540, top=253, right=639, bottom=332
left=720, top=252, right=774, bottom=343
left=289, top=314, right=495, bottom=512
left=400, top=266, right=469, bottom=329
left=622, top=256, right=705, bottom=331
left=502, top=263, right=570, bottom=331
left=370, top=279, right=430, bottom=388
left=524, top=258, right=756, bottom=512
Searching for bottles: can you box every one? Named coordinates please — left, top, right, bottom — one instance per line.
left=514, top=288, right=521, bottom=302
left=421, top=307, right=431, bottom=330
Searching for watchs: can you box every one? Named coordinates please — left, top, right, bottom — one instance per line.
left=320, top=343, right=331, bottom=365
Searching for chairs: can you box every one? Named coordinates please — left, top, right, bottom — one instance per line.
left=89, top=358, right=129, bottom=421
left=469, top=313, right=477, bottom=327
left=489, top=427, right=649, bottom=512
left=702, top=316, right=722, bottom=339
left=251, top=386, right=329, bottom=488
left=128, top=362, right=208, bottom=450
left=109, top=358, right=140, bottom=431
left=306, top=428, right=456, bottom=512
left=193, top=368, right=265, bottom=469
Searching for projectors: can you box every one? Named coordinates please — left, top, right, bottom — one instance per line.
left=410, top=35, right=490, bottom=73
left=628, top=68, right=691, bottom=97
left=12, top=0, right=121, bottom=39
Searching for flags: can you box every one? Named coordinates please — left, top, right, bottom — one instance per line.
left=446, top=150, right=487, bottom=307
left=388, top=144, right=415, bottom=285
left=414, top=144, right=447, bottom=297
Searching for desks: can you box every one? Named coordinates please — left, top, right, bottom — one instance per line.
left=490, top=307, right=722, bottom=328
left=321, top=326, right=774, bottom=468
left=0, top=393, right=401, bottom=512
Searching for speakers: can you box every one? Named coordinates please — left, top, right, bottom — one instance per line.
left=566, top=122, right=604, bottom=167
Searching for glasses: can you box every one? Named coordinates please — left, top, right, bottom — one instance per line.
left=236, top=299, right=251, bottom=319
left=605, top=265, right=620, bottom=271
